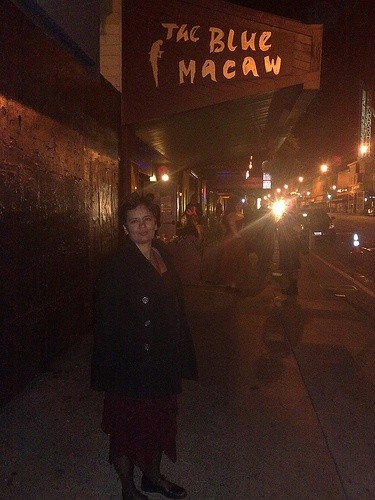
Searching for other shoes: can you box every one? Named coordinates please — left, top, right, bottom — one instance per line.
left=141, top=472, right=187, bottom=499
left=122, top=483, right=148, bottom=499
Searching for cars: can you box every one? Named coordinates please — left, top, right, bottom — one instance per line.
left=295, top=209, right=337, bottom=241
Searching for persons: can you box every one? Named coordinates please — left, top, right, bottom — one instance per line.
left=177, top=193, right=307, bottom=296
left=89, top=195, right=200, bottom=500
